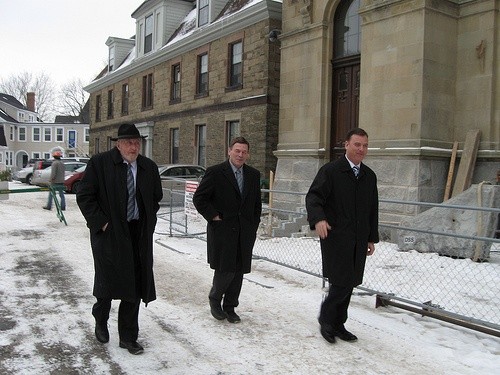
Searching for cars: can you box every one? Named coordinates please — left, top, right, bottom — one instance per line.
left=16, top=157, right=208, bottom=194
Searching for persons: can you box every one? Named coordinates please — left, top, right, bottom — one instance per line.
left=77, top=123, right=163, bottom=355
left=192, top=137, right=262, bottom=324
left=305, top=128, right=379, bottom=344
left=41, top=151, right=66, bottom=211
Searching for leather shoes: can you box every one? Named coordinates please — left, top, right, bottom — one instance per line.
left=336, top=325, right=357, bottom=342
left=223, top=306, right=241, bottom=323
left=209, top=295, right=226, bottom=320
left=119, top=339, right=143, bottom=355
left=318, top=317, right=335, bottom=343
left=95, top=317, right=109, bottom=343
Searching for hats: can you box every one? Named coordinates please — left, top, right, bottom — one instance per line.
left=110, top=123, right=148, bottom=141
left=52, top=152, right=61, bottom=156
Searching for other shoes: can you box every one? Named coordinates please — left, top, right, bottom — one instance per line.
left=42, top=206, right=51, bottom=210
left=59, top=207, right=65, bottom=210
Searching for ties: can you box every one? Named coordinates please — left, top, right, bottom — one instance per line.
left=353, top=166, right=360, bottom=180
left=235, top=170, right=243, bottom=194
left=127, top=162, right=135, bottom=222
